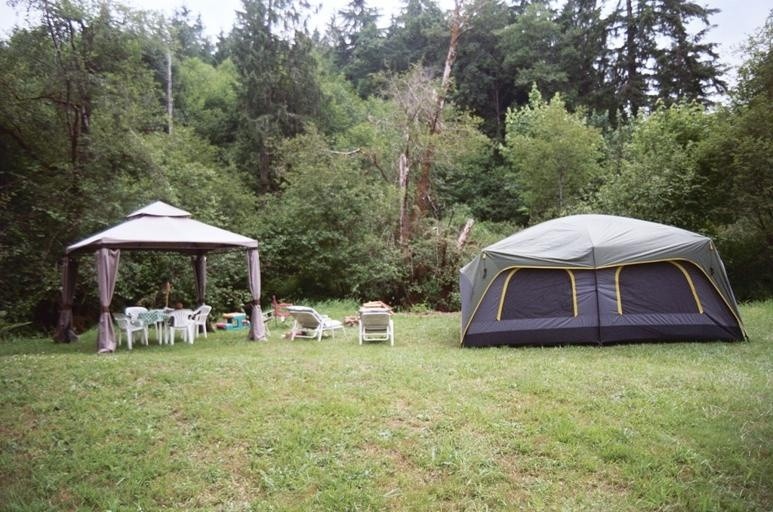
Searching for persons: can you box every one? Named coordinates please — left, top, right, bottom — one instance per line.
left=155, top=281, right=171, bottom=308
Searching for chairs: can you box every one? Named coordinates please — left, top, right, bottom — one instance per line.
left=286, top=305, right=346, bottom=341
left=358, top=305, right=394, bottom=348
left=112, top=305, right=211, bottom=350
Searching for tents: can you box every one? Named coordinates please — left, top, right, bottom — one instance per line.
left=459, top=213, right=748, bottom=345
